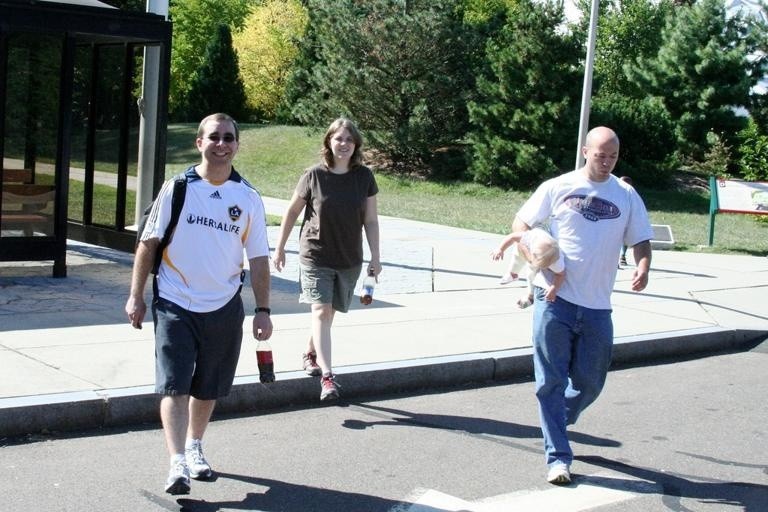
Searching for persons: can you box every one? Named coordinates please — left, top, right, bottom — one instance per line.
left=273, top=119, right=382, bottom=403
left=512, top=126, right=653, bottom=485
left=125, top=112, right=272, bottom=495
left=618, top=175, right=633, bottom=265
left=491, top=226, right=566, bottom=309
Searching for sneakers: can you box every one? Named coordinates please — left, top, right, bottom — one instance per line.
left=303, top=350, right=321, bottom=376
left=320, top=372, right=340, bottom=400
left=548, top=462, right=572, bottom=483
left=164, top=461, right=191, bottom=495
left=186, top=440, right=212, bottom=479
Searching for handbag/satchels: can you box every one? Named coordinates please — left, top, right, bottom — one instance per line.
left=135, top=174, right=186, bottom=273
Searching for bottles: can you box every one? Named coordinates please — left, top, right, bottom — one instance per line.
left=255, top=331, right=276, bottom=385
left=360, top=266, right=376, bottom=306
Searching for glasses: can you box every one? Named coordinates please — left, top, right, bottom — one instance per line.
left=208, top=133, right=234, bottom=143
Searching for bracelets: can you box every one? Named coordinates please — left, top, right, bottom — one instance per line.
left=254, top=307, right=271, bottom=315
